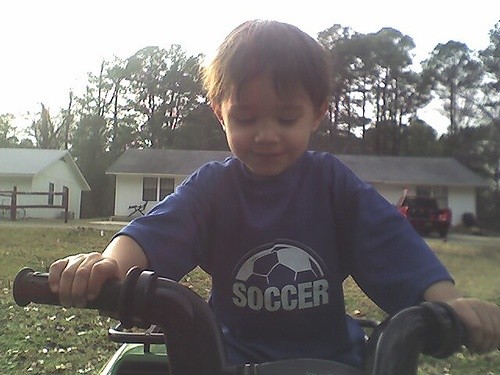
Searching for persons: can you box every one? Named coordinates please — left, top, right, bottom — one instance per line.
left=49, top=20, right=500, bottom=375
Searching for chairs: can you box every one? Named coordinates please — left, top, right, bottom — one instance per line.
left=128, top=200, right=148, bottom=217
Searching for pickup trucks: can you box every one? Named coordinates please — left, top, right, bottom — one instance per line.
left=395, top=195, right=452, bottom=238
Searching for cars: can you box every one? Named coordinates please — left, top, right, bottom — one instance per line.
left=12, top=267, right=500, bottom=375
